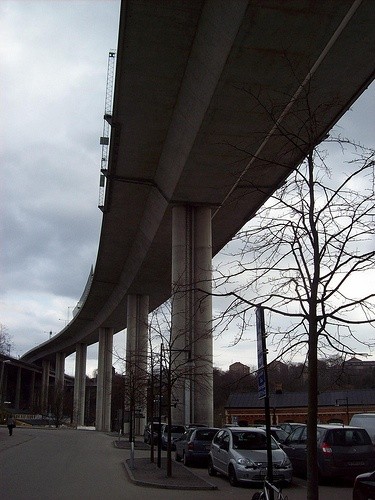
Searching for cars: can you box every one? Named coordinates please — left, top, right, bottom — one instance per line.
left=208, top=426, right=292, bottom=487
left=258, top=422, right=308, bottom=446
left=143, top=422, right=168, bottom=446
left=175, top=426, right=220, bottom=466
left=161, top=424, right=187, bottom=451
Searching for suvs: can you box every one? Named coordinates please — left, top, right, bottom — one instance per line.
left=280, top=424, right=374, bottom=487
